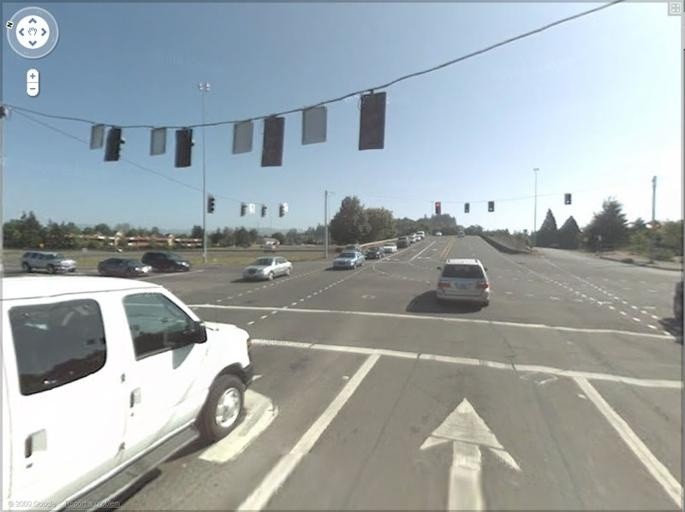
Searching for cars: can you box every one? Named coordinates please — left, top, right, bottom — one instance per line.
left=435, top=232, right=442, bottom=237
left=673, top=282, right=684, bottom=320
left=458, top=231, right=464, bottom=237
left=332, top=231, right=426, bottom=269
left=142, top=250, right=191, bottom=274
left=97, top=258, right=150, bottom=278
left=243, top=256, right=294, bottom=283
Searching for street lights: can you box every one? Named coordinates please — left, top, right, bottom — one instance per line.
left=531, top=166, right=540, bottom=250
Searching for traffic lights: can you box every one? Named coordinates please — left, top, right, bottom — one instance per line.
left=278, top=206, right=285, bottom=217
left=210, top=197, right=214, bottom=212
left=263, top=207, right=266, bottom=216
left=434, top=202, right=440, bottom=214
left=464, top=203, right=469, bottom=213
left=565, top=194, right=570, bottom=203
left=242, top=206, right=247, bottom=215
left=488, top=201, right=494, bottom=212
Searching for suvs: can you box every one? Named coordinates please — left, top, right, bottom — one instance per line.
left=19, top=250, right=77, bottom=275
left=1, top=274, right=250, bottom=512
left=435, top=257, right=491, bottom=305
